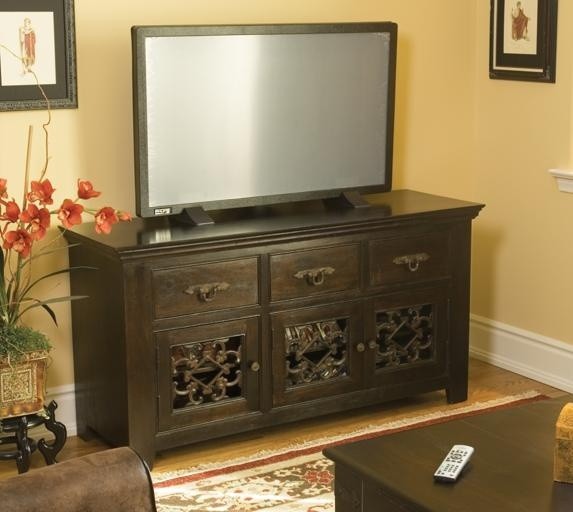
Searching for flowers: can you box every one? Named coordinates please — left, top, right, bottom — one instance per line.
left=1, top=45, right=132, bottom=369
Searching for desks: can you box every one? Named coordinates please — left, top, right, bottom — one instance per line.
left=323, top=391, right=573, bottom=512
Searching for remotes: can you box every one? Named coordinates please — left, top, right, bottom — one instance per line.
left=434, top=444, right=475, bottom=482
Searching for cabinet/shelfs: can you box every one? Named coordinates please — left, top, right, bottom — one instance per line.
left=0, top=400, right=67, bottom=474
left=57, top=188, right=487, bottom=472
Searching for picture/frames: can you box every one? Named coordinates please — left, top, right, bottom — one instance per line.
left=489, top=0, right=558, bottom=84
left=0, top=0, right=78, bottom=111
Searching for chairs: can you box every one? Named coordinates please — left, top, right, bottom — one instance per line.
left=0, top=446, right=157, bottom=512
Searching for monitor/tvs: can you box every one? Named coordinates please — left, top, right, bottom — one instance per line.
left=131, top=21, right=398, bottom=227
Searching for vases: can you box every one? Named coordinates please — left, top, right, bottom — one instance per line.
left=0, top=346, right=54, bottom=420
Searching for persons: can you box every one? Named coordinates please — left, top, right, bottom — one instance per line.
left=511, top=1, right=530, bottom=42
left=18, top=17, right=35, bottom=74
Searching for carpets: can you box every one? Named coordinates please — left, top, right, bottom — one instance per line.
left=149, top=391, right=551, bottom=512
